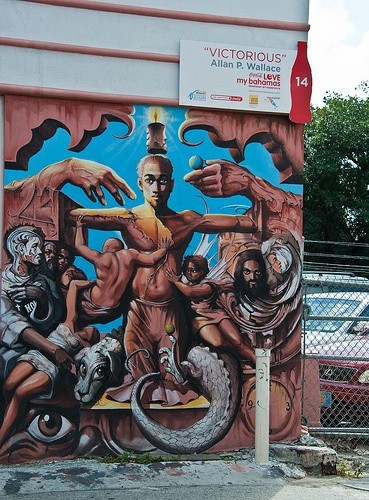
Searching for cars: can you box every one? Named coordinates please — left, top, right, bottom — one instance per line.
left=301, top=291, right=369, bottom=349
left=302, top=330, right=369, bottom=429
